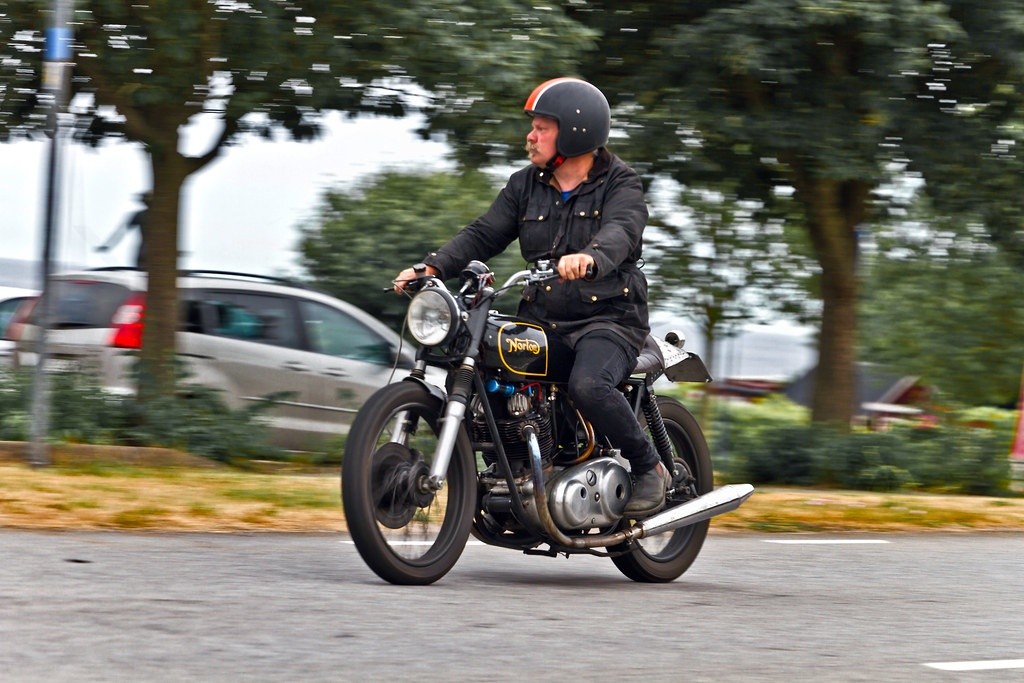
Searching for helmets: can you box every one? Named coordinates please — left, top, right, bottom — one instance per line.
left=523, top=77, right=611, bottom=157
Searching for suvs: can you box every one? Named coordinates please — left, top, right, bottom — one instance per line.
left=12, top=266, right=449, bottom=465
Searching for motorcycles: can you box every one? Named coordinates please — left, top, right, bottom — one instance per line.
left=339, top=258, right=756, bottom=587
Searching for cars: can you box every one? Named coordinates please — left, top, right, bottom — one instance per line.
left=0, top=286, right=40, bottom=388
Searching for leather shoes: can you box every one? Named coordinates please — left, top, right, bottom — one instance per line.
left=622, top=460, right=672, bottom=518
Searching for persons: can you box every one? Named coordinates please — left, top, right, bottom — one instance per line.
left=393, top=78, right=671, bottom=517
